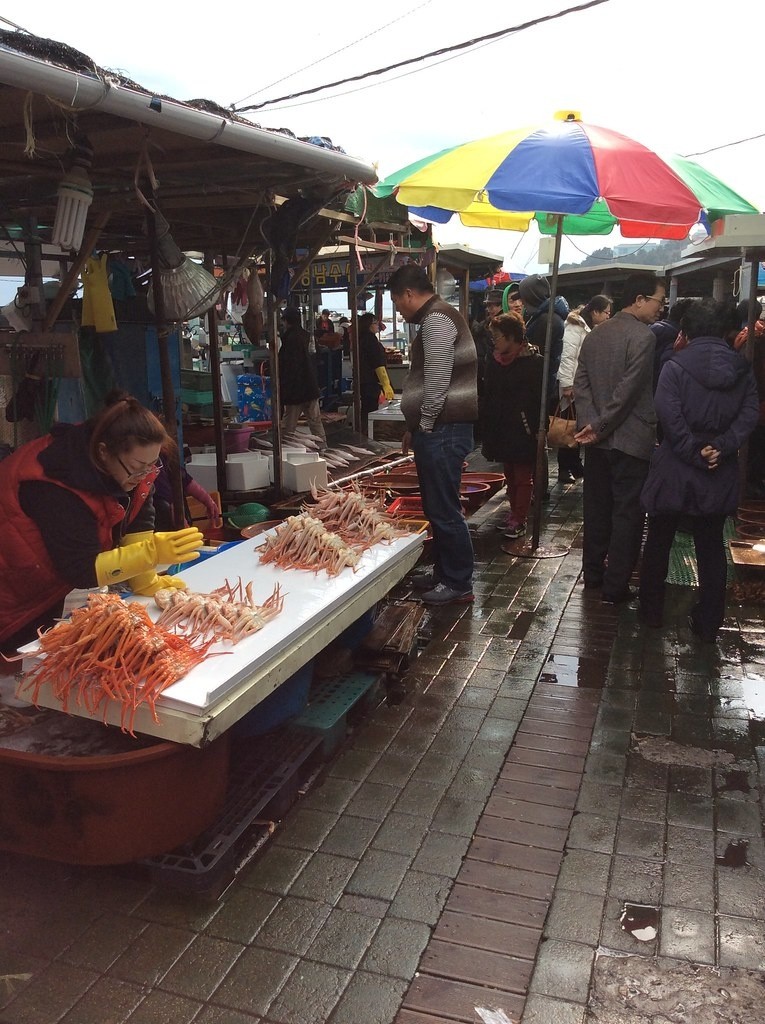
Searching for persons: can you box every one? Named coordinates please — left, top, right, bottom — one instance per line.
left=278, top=306, right=394, bottom=451
left=471, top=273, right=765, bottom=642
left=0, top=386, right=223, bottom=653
left=388, top=264, right=477, bottom=605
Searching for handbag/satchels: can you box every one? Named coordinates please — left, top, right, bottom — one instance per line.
left=547, top=395, right=579, bottom=449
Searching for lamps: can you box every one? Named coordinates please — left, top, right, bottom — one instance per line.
left=49, top=146, right=94, bottom=254
left=145, top=195, right=222, bottom=322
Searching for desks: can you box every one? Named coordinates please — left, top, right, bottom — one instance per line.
left=368, top=400, right=405, bottom=450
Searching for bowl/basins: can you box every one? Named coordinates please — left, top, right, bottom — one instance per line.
left=736, top=524, right=765, bottom=540
left=344, top=461, right=506, bottom=508
left=239, top=520, right=285, bottom=539
left=737, top=500, right=765, bottom=512
left=737, top=512, right=765, bottom=524
left=0, top=654, right=230, bottom=869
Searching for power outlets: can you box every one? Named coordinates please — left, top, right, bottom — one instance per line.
left=17, top=286, right=41, bottom=306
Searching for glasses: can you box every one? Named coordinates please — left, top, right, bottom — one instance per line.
left=642, top=294, right=666, bottom=306
left=602, top=310, right=611, bottom=316
left=372, top=320, right=379, bottom=326
left=113, top=452, right=163, bottom=481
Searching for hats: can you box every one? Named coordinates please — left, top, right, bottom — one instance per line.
left=280, top=306, right=299, bottom=322
left=483, top=289, right=503, bottom=303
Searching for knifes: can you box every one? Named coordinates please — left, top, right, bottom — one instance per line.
left=731, top=543, right=765, bottom=552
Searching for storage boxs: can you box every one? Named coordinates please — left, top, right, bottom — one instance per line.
left=180, top=368, right=223, bottom=392
left=282, top=459, right=327, bottom=492
left=187, top=403, right=213, bottom=418
left=224, top=458, right=270, bottom=491
left=268, top=455, right=274, bottom=483
left=186, top=462, right=217, bottom=493
left=181, top=388, right=213, bottom=404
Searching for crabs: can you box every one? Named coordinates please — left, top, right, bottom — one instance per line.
left=254, top=473, right=415, bottom=579
left=1, top=575, right=291, bottom=738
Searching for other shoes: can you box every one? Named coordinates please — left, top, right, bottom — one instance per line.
left=638, top=607, right=663, bottom=628
left=601, top=585, right=637, bottom=605
left=584, top=567, right=607, bottom=588
left=689, top=616, right=714, bottom=639
left=558, top=475, right=576, bottom=483
left=744, top=480, right=765, bottom=499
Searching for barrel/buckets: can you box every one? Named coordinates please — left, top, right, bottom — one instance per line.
left=217, top=540, right=246, bottom=552
left=166, top=551, right=218, bottom=576
left=224, top=427, right=255, bottom=453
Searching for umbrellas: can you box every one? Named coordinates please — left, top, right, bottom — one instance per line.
left=366, top=109, right=758, bottom=548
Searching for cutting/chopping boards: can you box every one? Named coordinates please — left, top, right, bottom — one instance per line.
left=729, top=540, right=765, bottom=566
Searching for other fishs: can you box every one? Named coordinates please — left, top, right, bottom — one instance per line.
left=250, top=429, right=376, bottom=468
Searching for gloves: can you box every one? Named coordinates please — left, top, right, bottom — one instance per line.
left=95, top=527, right=204, bottom=588
left=184, top=479, right=219, bottom=524
left=169, top=502, right=189, bottom=530
left=117, top=530, right=186, bottom=597
left=375, top=366, right=394, bottom=401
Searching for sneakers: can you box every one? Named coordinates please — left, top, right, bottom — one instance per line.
left=496, top=511, right=515, bottom=529
left=504, top=517, right=527, bottom=538
left=421, top=582, right=473, bottom=605
left=412, top=566, right=445, bottom=589
